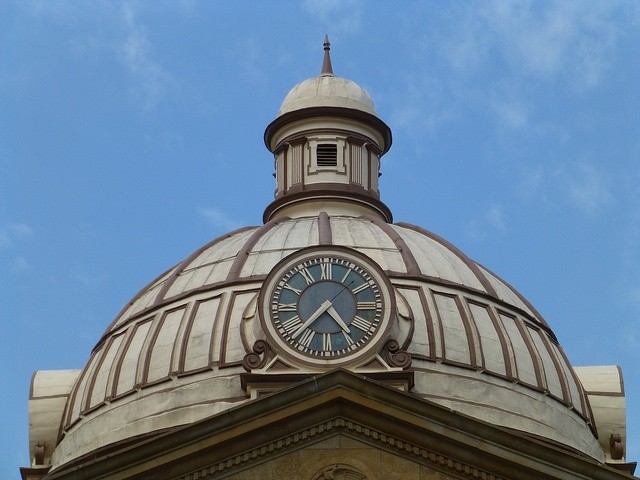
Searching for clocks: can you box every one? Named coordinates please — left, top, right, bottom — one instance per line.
left=258, top=243, right=395, bottom=370
